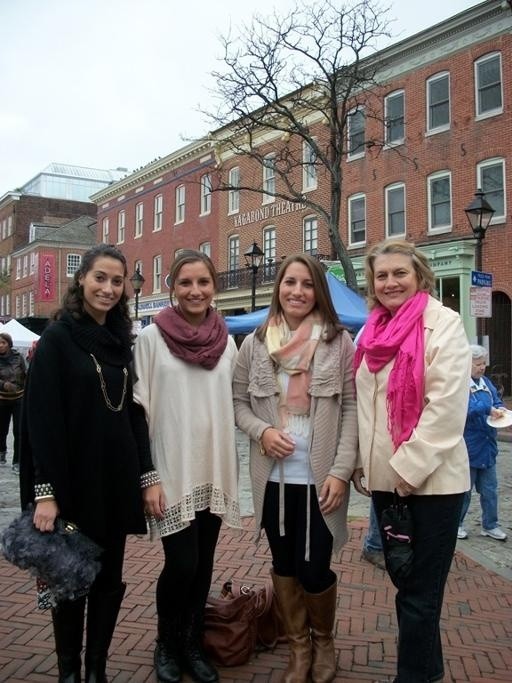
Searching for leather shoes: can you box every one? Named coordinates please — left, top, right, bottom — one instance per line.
left=154, top=645, right=219, bottom=683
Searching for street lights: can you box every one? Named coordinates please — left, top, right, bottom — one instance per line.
left=131, top=261, right=147, bottom=319
left=243, top=236, right=264, bottom=313
left=464, top=186, right=497, bottom=345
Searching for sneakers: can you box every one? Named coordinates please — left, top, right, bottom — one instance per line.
left=11, top=464, right=20, bottom=474
left=458, top=530, right=468, bottom=540
left=481, top=527, right=507, bottom=540
left=361, top=549, right=386, bottom=568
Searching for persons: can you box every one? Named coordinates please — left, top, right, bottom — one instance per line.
left=132, top=248, right=242, bottom=683
left=350, top=236, right=471, bottom=683
left=231, top=252, right=359, bottom=683
left=18, top=243, right=155, bottom=682
left=0, top=332, right=26, bottom=472
left=457, top=345, right=507, bottom=542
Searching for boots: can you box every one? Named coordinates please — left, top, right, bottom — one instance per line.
left=52, top=596, right=84, bottom=683
left=268, top=568, right=337, bottom=682
left=85, top=582, right=126, bottom=683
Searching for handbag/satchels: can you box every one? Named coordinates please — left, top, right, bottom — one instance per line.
left=381, top=507, right=414, bottom=588
left=201, top=583, right=282, bottom=669
left=1, top=506, right=100, bottom=609
